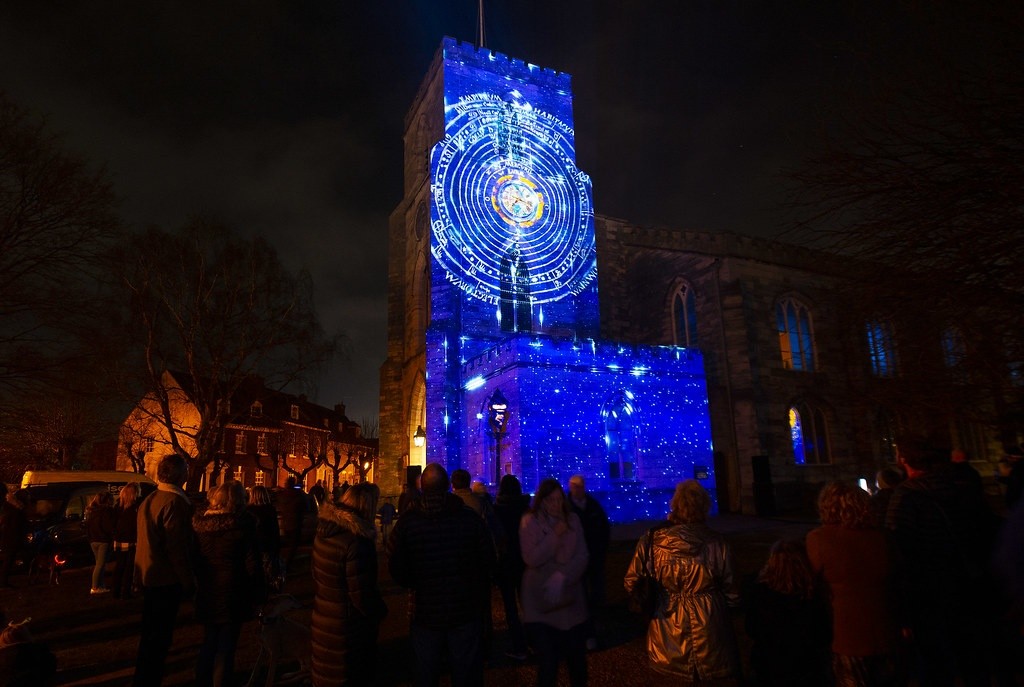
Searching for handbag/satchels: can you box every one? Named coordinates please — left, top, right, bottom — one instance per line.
left=628, top=529, right=654, bottom=615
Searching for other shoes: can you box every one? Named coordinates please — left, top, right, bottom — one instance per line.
left=505, top=649, right=526, bottom=660
left=586, top=636, right=597, bottom=650
left=90, top=587, right=110, bottom=594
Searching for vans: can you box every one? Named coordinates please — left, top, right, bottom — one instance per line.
left=21, top=470, right=157, bottom=545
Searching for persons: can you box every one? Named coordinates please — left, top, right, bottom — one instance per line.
left=0, top=417, right=1024, bottom=687
